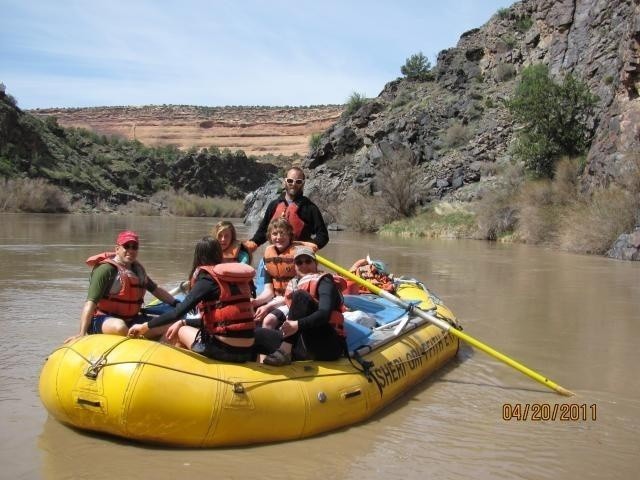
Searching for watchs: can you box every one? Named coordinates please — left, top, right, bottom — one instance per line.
left=173, top=298, right=181, bottom=307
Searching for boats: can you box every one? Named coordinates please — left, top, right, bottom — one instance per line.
left=33, top=276, right=468, bottom=453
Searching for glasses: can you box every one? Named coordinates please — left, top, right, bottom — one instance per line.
left=123, top=243, right=138, bottom=250
left=294, top=258, right=314, bottom=266
left=285, top=178, right=305, bottom=185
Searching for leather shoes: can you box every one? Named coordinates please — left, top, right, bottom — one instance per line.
left=263, top=348, right=292, bottom=366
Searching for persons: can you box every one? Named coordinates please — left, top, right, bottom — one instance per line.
left=62, top=230, right=181, bottom=345
left=250, top=218, right=318, bottom=330
left=242, top=167, right=329, bottom=253
left=255, top=248, right=347, bottom=367
left=127, top=237, right=256, bottom=363
left=211, top=220, right=253, bottom=266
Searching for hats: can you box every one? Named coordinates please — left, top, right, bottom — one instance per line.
left=117, top=231, right=138, bottom=245
left=294, top=247, right=316, bottom=261
